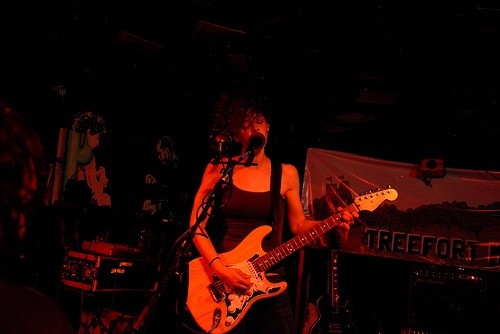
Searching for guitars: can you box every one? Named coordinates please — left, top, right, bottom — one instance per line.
left=309, top=231, right=358, bottom=334
left=186, top=185, right=399, bottom=334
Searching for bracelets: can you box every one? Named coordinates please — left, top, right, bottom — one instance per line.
left=207, top=258, right=222, bottom=268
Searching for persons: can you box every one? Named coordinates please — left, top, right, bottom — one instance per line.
left=188, top=96, right=359, bottom=334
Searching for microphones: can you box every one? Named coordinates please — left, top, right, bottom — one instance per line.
left=235, top=132, right=267, bottom=162
